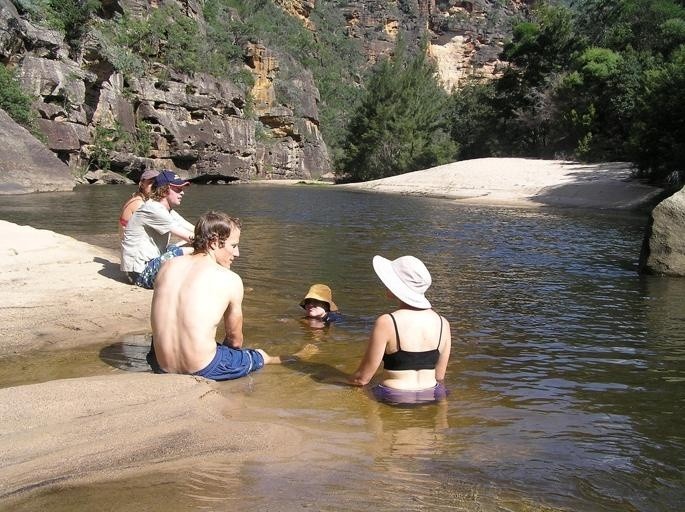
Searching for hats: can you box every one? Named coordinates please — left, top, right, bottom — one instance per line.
left=141, top=170, right=160, bottom=180
left=373, top=254, right=432, bottom=309
left=299, top=284, right=339, bottom=313
left=156, top=170, right=191, bottom=188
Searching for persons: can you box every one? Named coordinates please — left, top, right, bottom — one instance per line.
left=300, top=283, right=347, bottom=328
left=299, top=317, right=332, bottom=343
left=118, top=170, right=161, bottom=235
left=146, top=208, right=319, bottom=381
left=349, top=252, right=452, bottom=408
left=120, top=171, right=206, bottom=288
left=363, top=402, right=449, bottom=437
